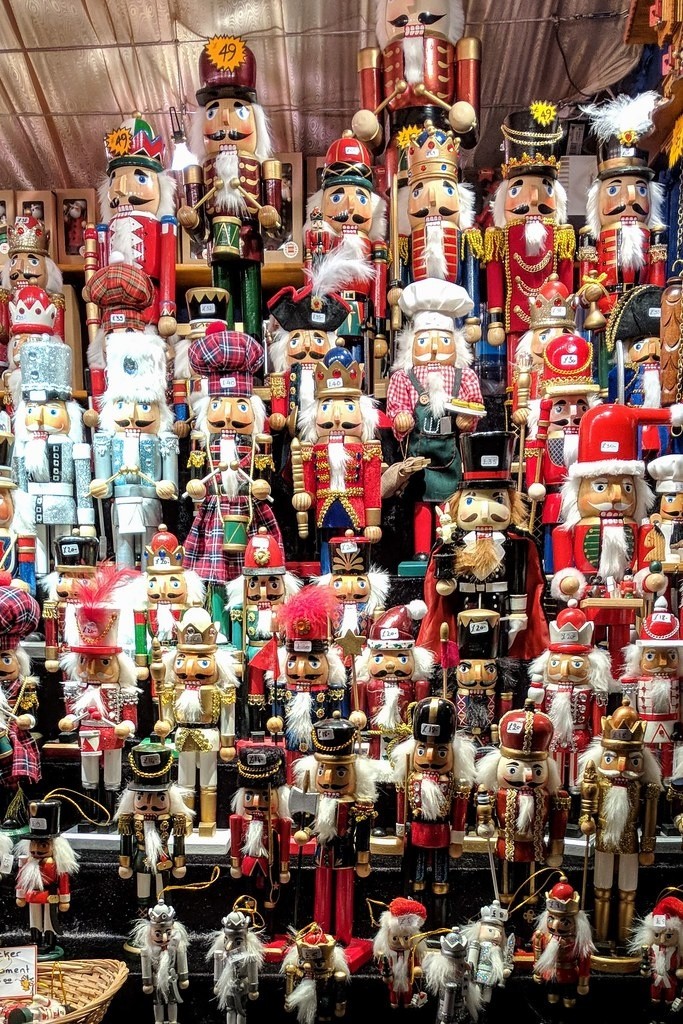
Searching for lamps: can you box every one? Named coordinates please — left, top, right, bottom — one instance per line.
left=168, top=107, right=198, bottom=171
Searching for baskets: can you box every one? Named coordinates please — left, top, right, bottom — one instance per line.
left=0, top=958, right=129, bottom=1024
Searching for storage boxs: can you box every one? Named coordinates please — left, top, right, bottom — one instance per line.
left=180, top=225, right=207, bottom=264
left=397, top=562, right=428, bottom=578
left=54, top=189, right=95, bottom=265
left=15, top=190, right=59, bottom=265
left=0, top=189, right=16, bottom=265
left=259, top=152, right=305, bottom=265
left=306, top=156, right=327, bottom=224
